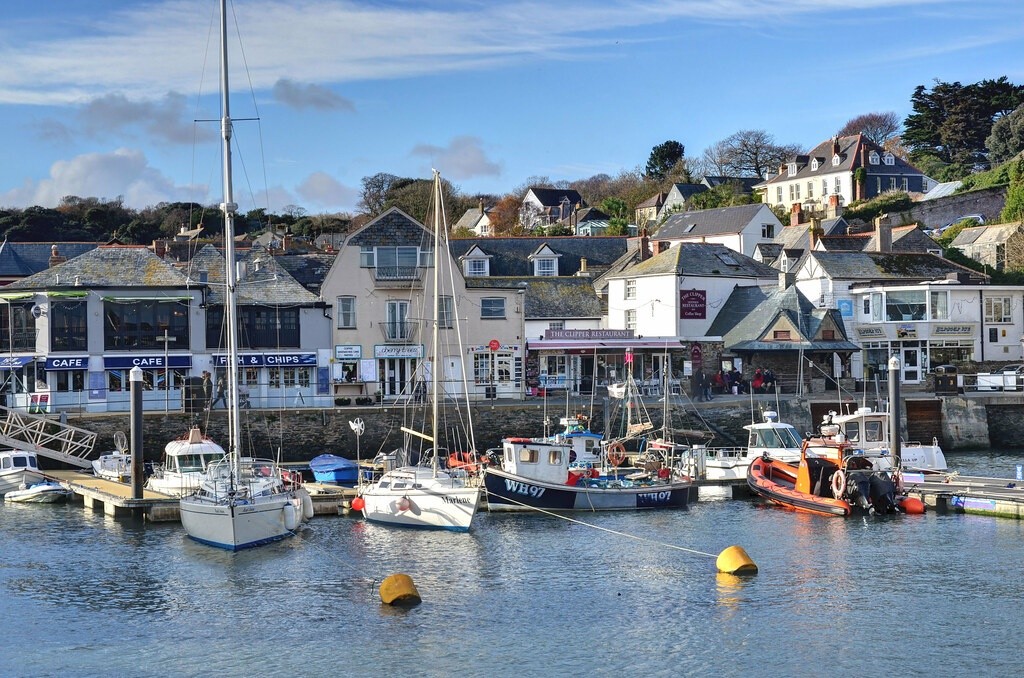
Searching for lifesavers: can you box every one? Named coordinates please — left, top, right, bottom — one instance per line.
left=505, top=436, right=532, bottom=443
left=607, top=441, right=626, bottom=465
left=832, top=469, right=847, bottom=497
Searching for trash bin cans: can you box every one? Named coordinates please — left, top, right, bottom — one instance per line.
left=181, top=376, right=205, bottom=413
left=934, top=365, right=958, bottom=396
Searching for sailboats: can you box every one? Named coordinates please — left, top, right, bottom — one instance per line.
left=178, top=1, right=317, bottom=554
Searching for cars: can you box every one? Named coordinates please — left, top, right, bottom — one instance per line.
left=993, top=363, right=1024, bottom=390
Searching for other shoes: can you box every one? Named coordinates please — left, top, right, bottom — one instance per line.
left=710, top=397, right=714, bottom=400
left=706, top=399, right=710, bottom=401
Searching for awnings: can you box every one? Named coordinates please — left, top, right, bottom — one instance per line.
left=528, top=342, right=685, bottom=349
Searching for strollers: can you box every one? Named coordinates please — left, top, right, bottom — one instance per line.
left=226, top=384, right=251, bottom=410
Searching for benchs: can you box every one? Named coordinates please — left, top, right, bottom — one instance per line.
left=771, top=374, right=811, bottom=393
left=709, top=385, right=722, bottom=395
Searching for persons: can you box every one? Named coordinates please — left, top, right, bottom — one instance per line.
left=713, top=367, right=747, bottom=394
left=752, top=368, right=772, bottom=394
left=201, top=371, right=212, bottom=410
left=211, top=375, right=228, bottom=410
left=692, top=367, right=714, bottom=402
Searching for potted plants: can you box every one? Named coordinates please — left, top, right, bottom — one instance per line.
left=375, top=387, right=385, bottom=403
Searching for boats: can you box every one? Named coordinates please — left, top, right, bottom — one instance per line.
left=3, top=481, right=63, bottom=502
left=90, top=451, right=131, bottom=482
left=747, top=422, right=925, bottom=517
left=144, top=428, right=228, bottom=497
left=357, top=447, right=451, bottom=483
left=0, top=448, right=46, bottom=495
left=483, top=352, right=949, bottom=512
left=448, top=451, right=490, bottom=473
left=309, top=454, right=371, bottom=484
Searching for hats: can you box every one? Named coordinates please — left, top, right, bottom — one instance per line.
left=756, top=369, right=761, bottom=373
